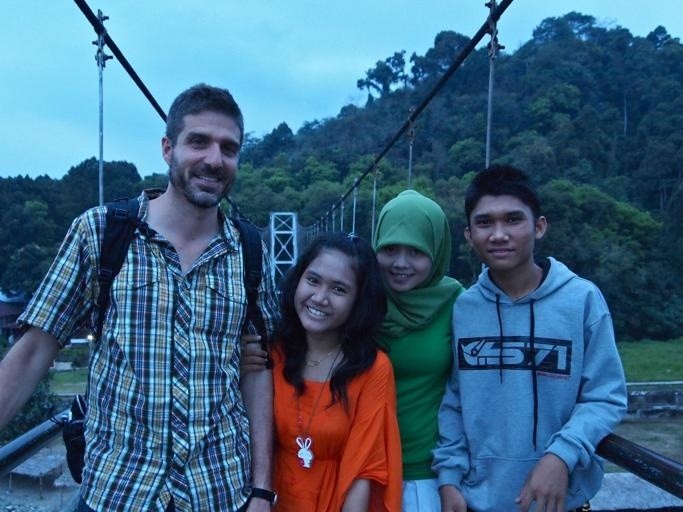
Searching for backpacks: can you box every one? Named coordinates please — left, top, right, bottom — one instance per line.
left=63, top=199, right=262, bottom=484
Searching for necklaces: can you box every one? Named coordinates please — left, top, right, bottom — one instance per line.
left=305, top=350, right=335, bottom=367
left=290, top=344, right=342, bottom=470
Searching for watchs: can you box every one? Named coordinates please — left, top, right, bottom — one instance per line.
left=251, top=488, right=278, bottom=506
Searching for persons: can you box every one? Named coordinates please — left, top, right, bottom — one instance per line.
left=238, top=231, right=405, bottom=512
left=430, top=164, right=629, bottom=512
left=0, top=80, right=281, bottom=512
left=365, top=189, right=468, bottom=511
